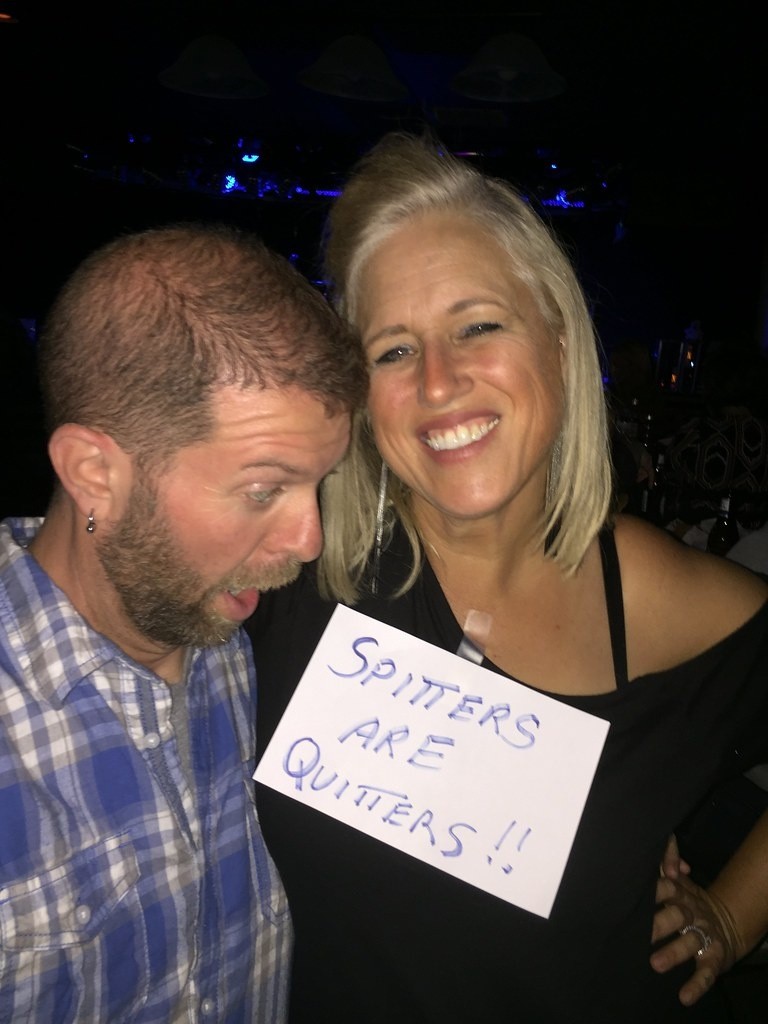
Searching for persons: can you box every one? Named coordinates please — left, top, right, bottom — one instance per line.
left=0, top=139, right=767, bottom=1023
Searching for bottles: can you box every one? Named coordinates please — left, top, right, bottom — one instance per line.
left=639, top=453, right=666, bottom=528
left=705, top=486, right=740, bottom=558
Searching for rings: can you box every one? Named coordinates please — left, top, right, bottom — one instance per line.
left=681, top=923, right=712, bottom=956
